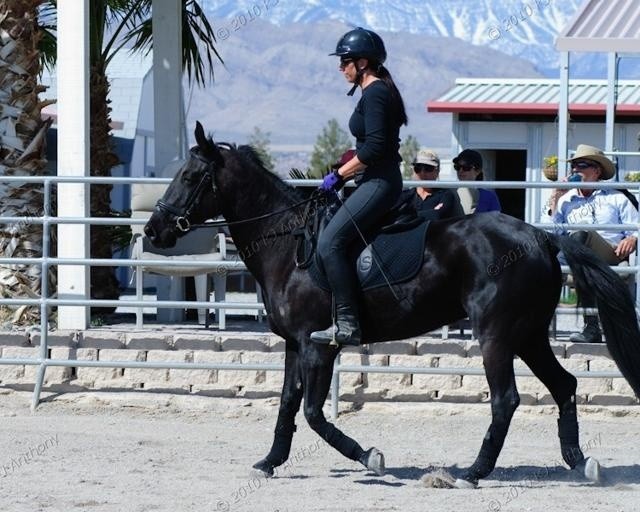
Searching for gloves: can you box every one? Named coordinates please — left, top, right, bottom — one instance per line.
left=318, top=169, right=342, bottom=195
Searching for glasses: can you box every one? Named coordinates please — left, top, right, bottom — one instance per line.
left=340, top=55, right=359, bottom=66
left=414, top=164, right=435, bottom=173
left=570, top=161, right=597, bottom=169
left=454, top=164, right=476, bottom=171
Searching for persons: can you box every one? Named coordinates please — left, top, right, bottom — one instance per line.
left=450, top=149, right=501, bottom=214
left=405, top=148, right=466, bottom=219
left=542, top=144, right=639, bottom=343
left=310, top=28, right=410, bottom=346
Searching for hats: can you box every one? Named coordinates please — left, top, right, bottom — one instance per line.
left=566, top=144, right=615, bottom=180
left=453, top=149, right=482, bottom=169
left=411, top=149, right=440, bottom=168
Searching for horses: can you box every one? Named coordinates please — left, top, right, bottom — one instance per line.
left=140, top=118, right=640, bottom=491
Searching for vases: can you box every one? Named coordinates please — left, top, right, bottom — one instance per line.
left=542, top=168, right=558, bottom=181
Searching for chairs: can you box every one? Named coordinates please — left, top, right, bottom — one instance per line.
left=131, top=183, right=263, bottom=330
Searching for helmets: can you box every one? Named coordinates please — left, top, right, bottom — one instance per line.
left=328, top=26, right=387, bottom=63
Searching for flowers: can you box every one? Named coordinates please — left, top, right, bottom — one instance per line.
left=542, top=155, right=558, bottom=168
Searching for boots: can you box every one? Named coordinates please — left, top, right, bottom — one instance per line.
left=310, top=255, right=362, bottom=346
left=570, top=315, right=602, bottom=343
